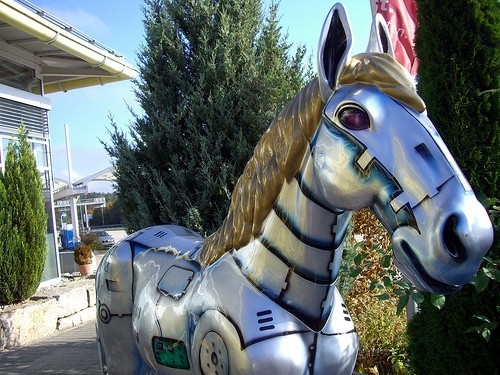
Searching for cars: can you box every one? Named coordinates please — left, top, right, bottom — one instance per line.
left=81, top=230, right=115, bottom=248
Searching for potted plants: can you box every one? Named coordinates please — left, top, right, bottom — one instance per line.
left=74, top=245, right=93, bottom=276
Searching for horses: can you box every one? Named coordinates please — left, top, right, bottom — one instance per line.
left=95, top=3, right=494, bottom=375
